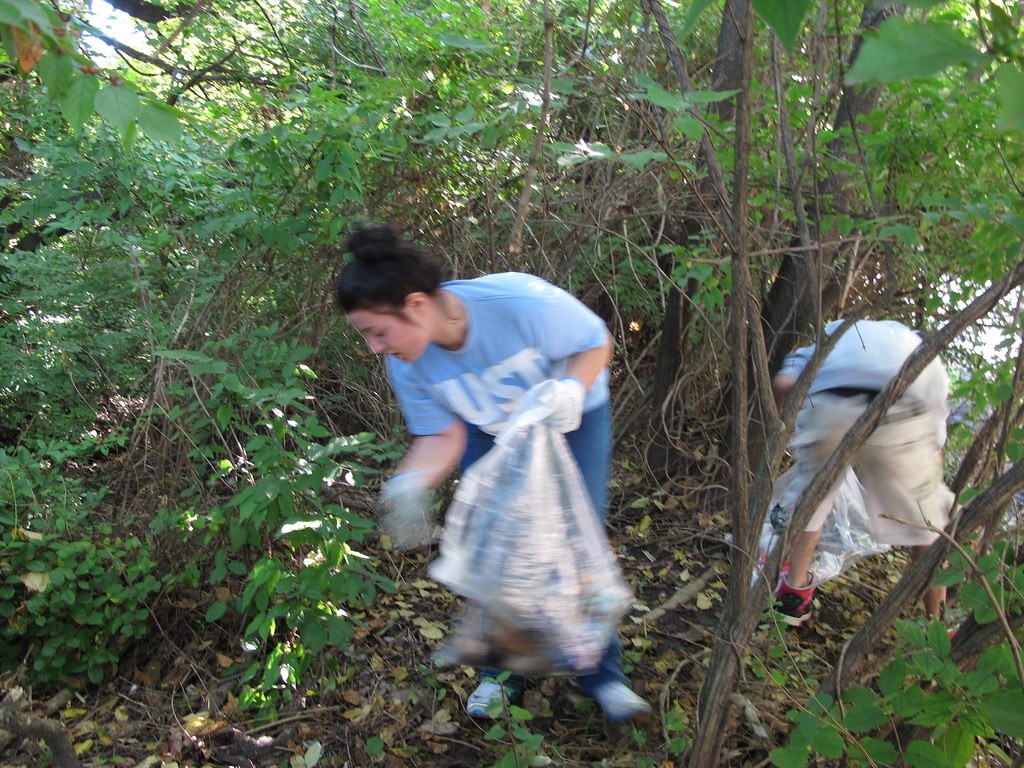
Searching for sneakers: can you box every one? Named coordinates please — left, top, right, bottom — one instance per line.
left=595, top=680, right=654, bottom=722
left=925, top=623, right=961, bottom=641
left=772, top=571, right=815, bottom=627
left=466, top=676, right=513, bottom=720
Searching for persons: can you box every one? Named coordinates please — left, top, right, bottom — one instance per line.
left=337, top=227, right=651, bottom=720
left=767, top=311, right=956, bottom=623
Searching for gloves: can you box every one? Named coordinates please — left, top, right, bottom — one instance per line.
left=548, top=376, right=586, bottom=433
